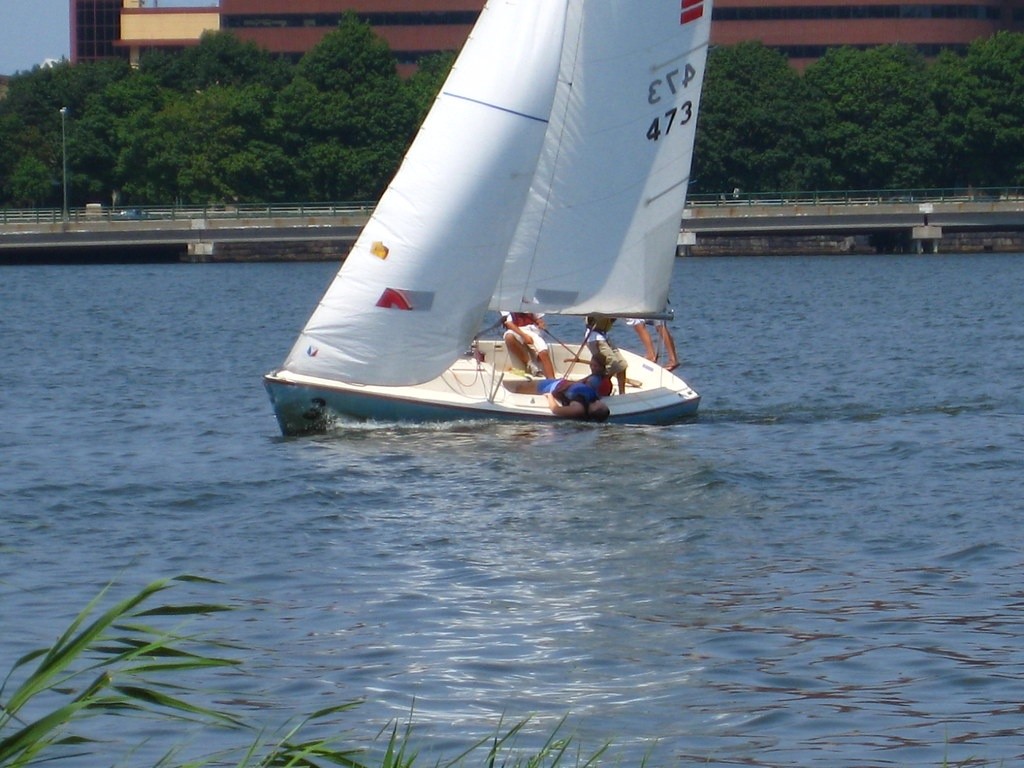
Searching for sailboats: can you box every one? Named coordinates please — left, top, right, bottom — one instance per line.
left=262, top=1, right=713, bottom=432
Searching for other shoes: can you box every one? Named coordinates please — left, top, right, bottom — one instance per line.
left=525, top=360, right=542, bottom=376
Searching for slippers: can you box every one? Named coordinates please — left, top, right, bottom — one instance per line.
left=663, top=362, right=680, bottom=371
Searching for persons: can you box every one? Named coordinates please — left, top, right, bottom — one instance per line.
left=500, top=295, right=682, bottom=422
left=732, top=185, right=740, bottom=200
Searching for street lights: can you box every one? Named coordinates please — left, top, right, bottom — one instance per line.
left=59, top=107, right=69, bottom=221
